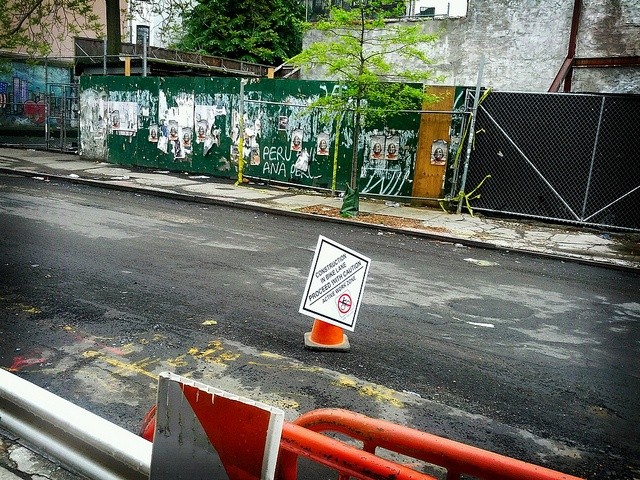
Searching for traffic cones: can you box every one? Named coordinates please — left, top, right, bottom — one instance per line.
left=304, top=319, right=351, bottom=352
